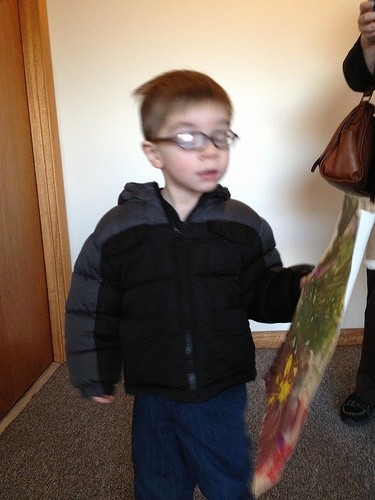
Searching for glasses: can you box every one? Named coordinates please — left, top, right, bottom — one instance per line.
left=149, top=128, right=239, bottom=152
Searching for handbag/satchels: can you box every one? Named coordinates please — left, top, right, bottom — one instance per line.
left=310, top=101, right=375, bottom=200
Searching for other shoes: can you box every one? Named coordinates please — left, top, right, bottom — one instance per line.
left=341, top=392, right=373, bottom=424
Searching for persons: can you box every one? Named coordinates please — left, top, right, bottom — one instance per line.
left=339, top=0, right=375, bottom=428
left=65, top=70, right=314, bottom=500
